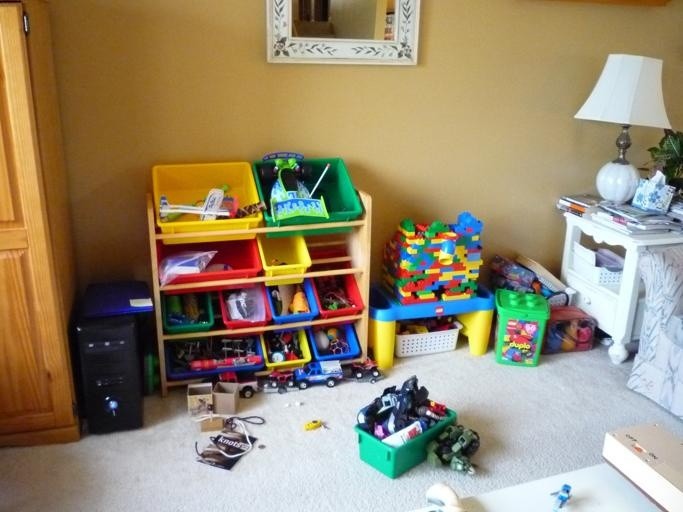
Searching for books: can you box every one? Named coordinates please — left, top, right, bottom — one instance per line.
left=556, top=188, right=683, bottom=238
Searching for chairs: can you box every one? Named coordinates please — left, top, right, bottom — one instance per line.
left=625, top=244, right=683, bottom=420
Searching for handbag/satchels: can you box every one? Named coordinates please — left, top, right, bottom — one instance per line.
left=540, top=305, right=598, bottom=354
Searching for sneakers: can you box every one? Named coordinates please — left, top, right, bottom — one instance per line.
left=425, top=483, right=464, bottom=512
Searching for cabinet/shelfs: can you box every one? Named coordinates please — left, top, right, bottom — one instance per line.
left=0, top=0, right=83, bottom=447
left=143, top=188, right=372, bottom=398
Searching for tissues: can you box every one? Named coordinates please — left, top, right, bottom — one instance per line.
left=631, top=170, right=676, bottom=216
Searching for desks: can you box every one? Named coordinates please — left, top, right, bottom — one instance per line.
left=558, top=210, right=683, bottom=364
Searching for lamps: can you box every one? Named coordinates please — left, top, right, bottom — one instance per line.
left=573, top=54, right=671, bottom=205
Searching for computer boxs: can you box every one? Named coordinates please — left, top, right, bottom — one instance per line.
left=74, top=313, right=143, bottom=433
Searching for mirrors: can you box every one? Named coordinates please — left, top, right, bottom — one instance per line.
left=264, top=0, right=422, bottom=67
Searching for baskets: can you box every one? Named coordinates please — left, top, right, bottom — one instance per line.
left=515, top=252, right=576, bottom=307
left=593, top=266, right=623, bottom=287
left=395, top=320, right=464, bottom=358
